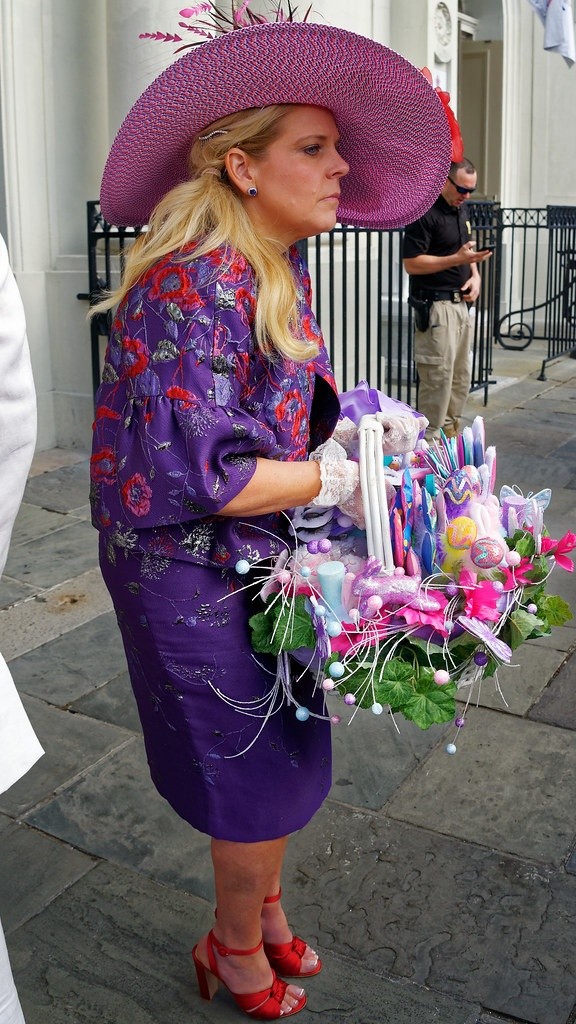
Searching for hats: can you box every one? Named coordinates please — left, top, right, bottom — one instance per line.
left=96, top=21, right=455, bottom=232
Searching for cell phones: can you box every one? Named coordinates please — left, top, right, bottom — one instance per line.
left=477, top=245, right=497, bottom=253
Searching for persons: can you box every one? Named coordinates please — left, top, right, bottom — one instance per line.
left=0, top=220, right=50, bottom=1022
left=87, top=53, right=393, bottom=1024
left=397, top=155, right=496, bottom=456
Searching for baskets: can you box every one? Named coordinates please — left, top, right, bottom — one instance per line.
left=311, top=413, right=491, bottom=705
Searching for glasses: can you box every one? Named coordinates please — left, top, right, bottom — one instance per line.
left=445, top=174, right=479, bottom=195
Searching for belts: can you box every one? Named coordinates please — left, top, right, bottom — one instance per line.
left=411, top=286, right=469, bottom=303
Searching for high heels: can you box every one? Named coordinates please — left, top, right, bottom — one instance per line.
left=189, top=927, right=308, bottom=1020
left=212, top=886, right=323, bottom=978
